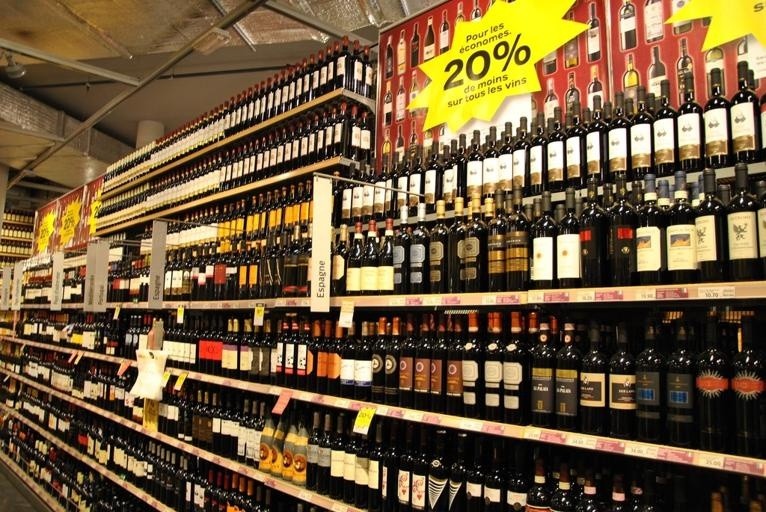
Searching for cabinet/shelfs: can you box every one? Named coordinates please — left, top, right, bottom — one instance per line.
left=0, top=162, right=766, bottom=512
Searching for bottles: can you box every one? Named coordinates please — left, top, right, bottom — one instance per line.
left=0, top=36, right=375, bottom=512
left=370, top=0, right=760, bottom=100
left=377, top=76, right=765, bottom=512
